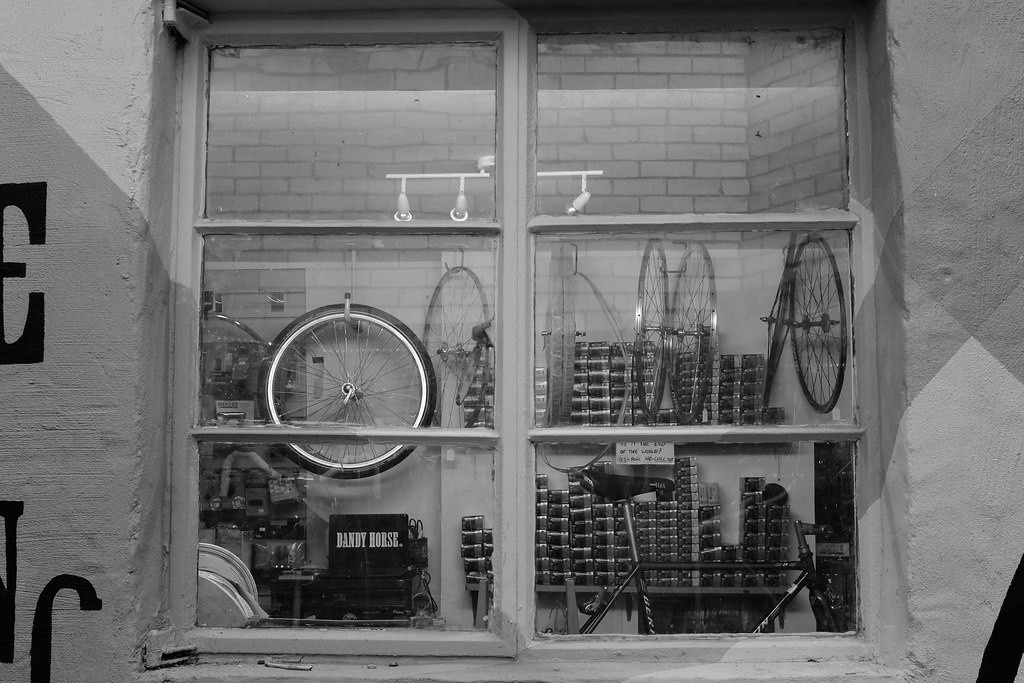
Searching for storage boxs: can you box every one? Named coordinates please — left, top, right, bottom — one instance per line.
left=461, top=341, right=791, bottom=588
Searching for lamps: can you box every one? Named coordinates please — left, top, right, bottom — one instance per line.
left=384, top=167, right=602, bottom=220
